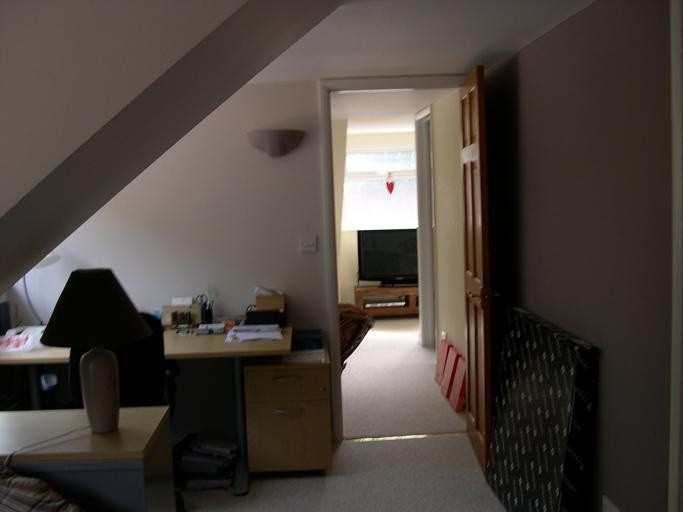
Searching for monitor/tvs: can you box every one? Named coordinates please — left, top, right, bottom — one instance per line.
left=357, top=229, right=418, bottom=287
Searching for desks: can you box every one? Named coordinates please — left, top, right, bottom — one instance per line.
left=0, top=322, right=293, bottom=495
left=0, top=405, right=176, bottom=512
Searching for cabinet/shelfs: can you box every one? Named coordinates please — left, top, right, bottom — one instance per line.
left=243, top=348, right=333, bottom=472
left=354, top=286, right=418, bottom=316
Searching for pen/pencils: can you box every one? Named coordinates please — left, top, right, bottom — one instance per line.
left=208, top=300, right=213, bottom=309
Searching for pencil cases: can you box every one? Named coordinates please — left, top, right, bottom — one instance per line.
left=197, top=323, right=225, bottom=334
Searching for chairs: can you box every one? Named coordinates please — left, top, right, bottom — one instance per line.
left=338, top=304, right=375, bottom=374
left=70, top=314, right=187, bottom=512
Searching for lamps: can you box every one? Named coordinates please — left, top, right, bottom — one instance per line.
left=246, top=129, right=304, bottom=156
left=41, top=269, right=153, bottom=433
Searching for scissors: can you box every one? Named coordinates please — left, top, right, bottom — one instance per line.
left=195, top=295, right=207, bottom=304
